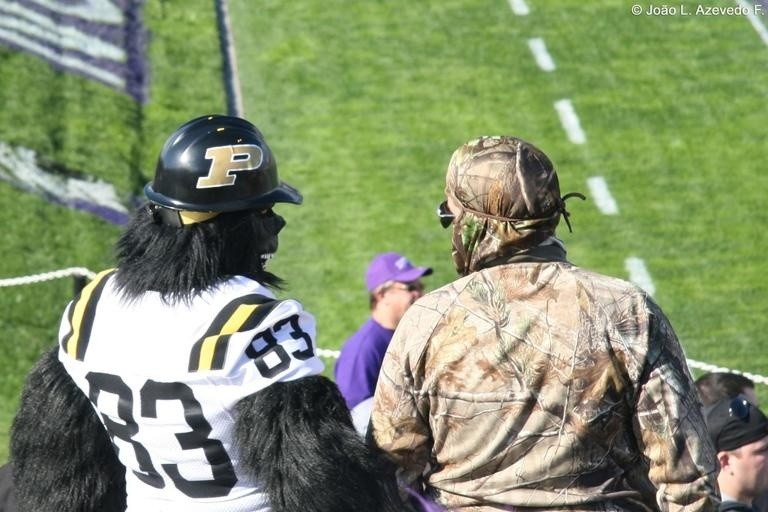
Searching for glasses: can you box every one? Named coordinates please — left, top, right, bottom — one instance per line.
left=728, top=397, right=750, bottom=422
left=437, top=200, right=457, bottom=228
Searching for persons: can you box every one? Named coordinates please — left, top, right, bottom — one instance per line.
left=690, top=370, right=759, bottom=416
left=332, top=253, right=434, bottom=509
left=364, top=132, right=722, bottom=511
left=702, top=396, right=766, bottom=512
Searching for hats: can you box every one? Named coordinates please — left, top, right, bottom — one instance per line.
left=705, top=397, right=768, bottom=452
left=365, top=252, right=433, bottom=292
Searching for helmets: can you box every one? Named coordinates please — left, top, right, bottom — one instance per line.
left=144, top=113, right=303, bottom=214
